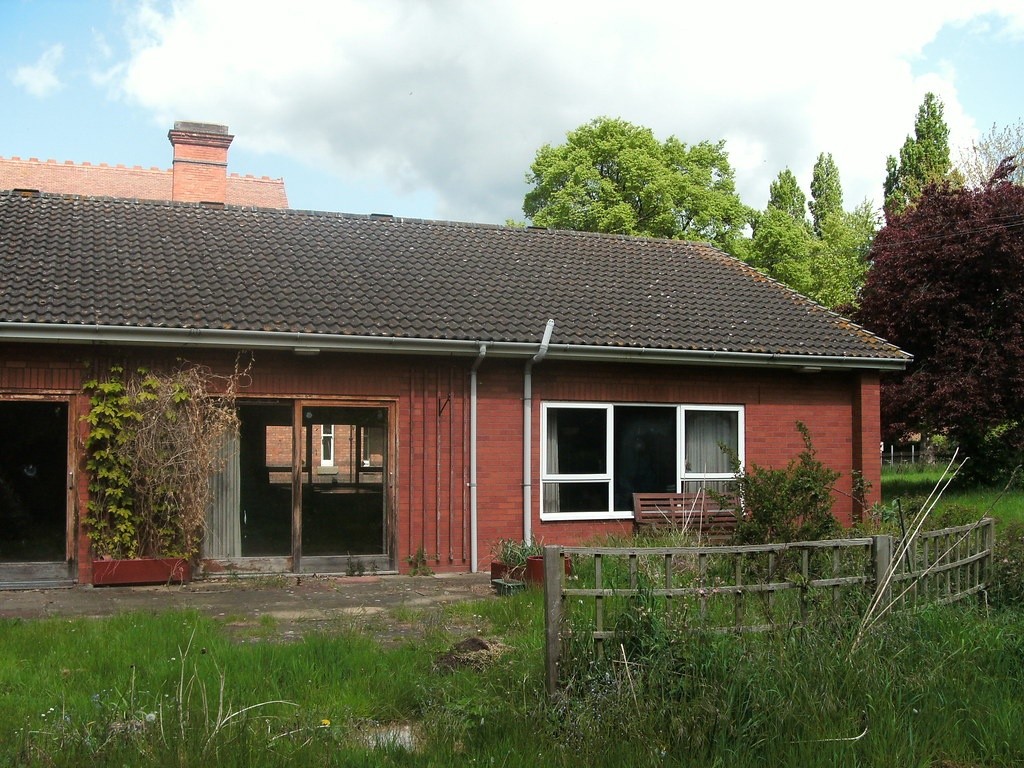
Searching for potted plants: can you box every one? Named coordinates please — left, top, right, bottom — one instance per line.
left=489, top=535, right=572, bottom=596
left=80, top=347, right=256, bottom=586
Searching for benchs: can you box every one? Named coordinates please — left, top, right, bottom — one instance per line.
left=631, top=492, right=741, bottom=546
